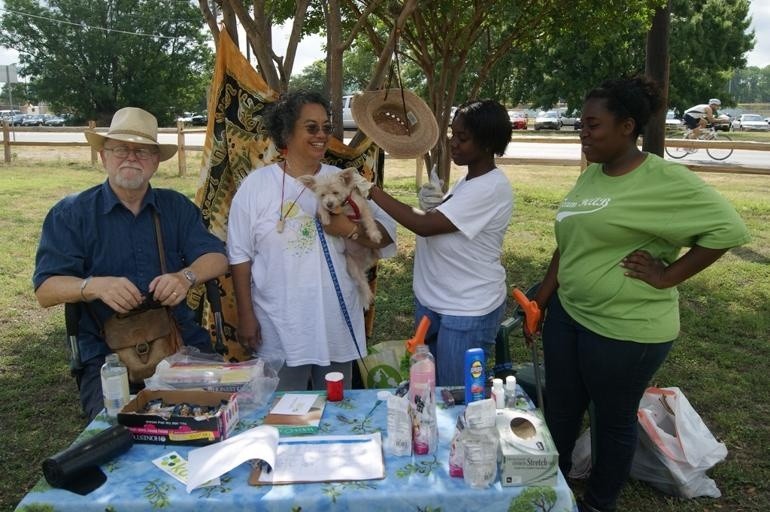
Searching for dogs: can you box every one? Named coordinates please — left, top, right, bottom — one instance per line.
left=297, top=166, right=384, bottom=313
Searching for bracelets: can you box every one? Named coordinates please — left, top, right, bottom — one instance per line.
left=80, top=275, right=91, bottom=301
left=347, top=225, right=360, bottom=242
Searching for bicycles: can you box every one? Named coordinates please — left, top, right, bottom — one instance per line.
left=665, top=117, right=735, bottom=161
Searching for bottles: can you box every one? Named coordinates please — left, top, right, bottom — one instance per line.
left=699, top=133, right=707, bottom=140
left=457, top=413, right=499, bottom=489
left=490, top=375, right=517, bottom=408
left=409, top=344, right=437, bottom=456
left=100, top=352, right=131, bottom=427
left=462, top=347, right=486, bottom=407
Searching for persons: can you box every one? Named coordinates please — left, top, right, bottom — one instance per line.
left=33, top=106, right=228, bottom=424
left=351, top=99, right=514, bottom=385
left=522, top=82, right=752, bottom=512
left=226, top=88, right=398, bottom=389
left=683, top=98, right=728, bottom=153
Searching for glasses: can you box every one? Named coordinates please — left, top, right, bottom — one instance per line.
left=103, top=146, right=159, bottom=160
left=293, top=125, right=336, bottom=135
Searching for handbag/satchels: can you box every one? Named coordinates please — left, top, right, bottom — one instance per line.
left=357, top=338, right=411, bottom=388
left=105, top=306, right=187, bottom=384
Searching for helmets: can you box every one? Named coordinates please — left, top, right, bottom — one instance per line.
left=709, top=99, right=722, bottom=105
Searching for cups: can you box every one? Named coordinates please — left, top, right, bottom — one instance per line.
left=324, top=372, right=344, bottom=402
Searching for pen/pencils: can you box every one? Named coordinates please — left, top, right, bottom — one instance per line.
left=267, top=463, right=272, bottom=473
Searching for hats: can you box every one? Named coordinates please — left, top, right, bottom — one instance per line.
left=84, top=107, right=178, bottom=162
left=351, top=87, right=440, bottom=159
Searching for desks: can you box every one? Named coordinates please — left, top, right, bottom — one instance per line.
left=13, top=385, right=578, bottom=511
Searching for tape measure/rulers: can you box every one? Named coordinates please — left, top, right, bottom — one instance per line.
left=279, top=439, right=371, bottom=445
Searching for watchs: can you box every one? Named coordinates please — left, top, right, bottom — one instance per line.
left=183, top=270, right=196, bottom=285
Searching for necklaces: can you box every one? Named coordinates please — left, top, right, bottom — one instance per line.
left=277, top=160, right=319, bottom=233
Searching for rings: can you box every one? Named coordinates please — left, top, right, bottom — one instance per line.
left=172, top=292, right=178, bottom=296
left=243, top=344, right=248, bottom=346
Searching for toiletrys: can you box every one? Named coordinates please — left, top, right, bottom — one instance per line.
left=505, top=375, right=516, bottom=407
left=491, top=378, right=505, bottom=408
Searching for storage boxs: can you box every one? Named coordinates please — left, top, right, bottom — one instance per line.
left=156, top=358, right=265, bottom=409
left=117, top=386, right=240, bottom=446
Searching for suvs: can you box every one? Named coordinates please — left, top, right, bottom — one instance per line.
left=559, top=107, right=582, bottom=128
left=710, top=109, right=730, bottom=132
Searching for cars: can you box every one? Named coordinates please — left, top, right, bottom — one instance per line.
left=343, top=94, right=365, bottom=129
left=1, top=106, right=74, bottom=127
left=507, top=111, right=529, bottom=130
left=533, top=111, right=562, bottom=130
left=666, top=109, right=682, bottom=129
left=441, top=104, right=459, bottom=127
left=731, top=113, right=769, bottom=132
left=173, top=113, right=207, bottom=125
